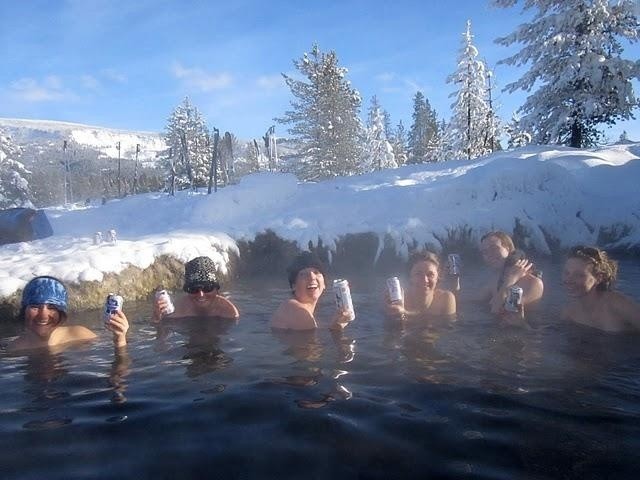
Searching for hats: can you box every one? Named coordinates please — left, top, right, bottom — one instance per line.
left=290, top=260, right=325, bottom=287
left=183, top=256, right=218, bottom=292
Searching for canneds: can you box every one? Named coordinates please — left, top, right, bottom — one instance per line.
left=95, top=232, right=102, bottom=244
left=448, top=254, right=460, bottom=275
left=504, top=286, right=523, bottom=313
left=332, top=279, right=355, bottom=321
left=385, top=276, right=402, bottom=305
left=103, top=293, right=122, bottom=326
left=155, top=290, right=175, bottom=316
left=109, top=230, right=116, bottom=243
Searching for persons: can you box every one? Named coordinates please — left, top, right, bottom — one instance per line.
left=479, top=293, right=544, bottom=355
left=152, top=315, right=240, bottom=374
left=499, top=243, right=640, bottom=333
left=478, top=229, right=545, bottom=315
left=266, top=252, right=351, bottom=331
left=386, top=313, right=455, bottom=380
left=7, top=337, right=129, bottom=404
left=268, top=324, right=353, bottom=385
left=148, top=256, right=241, bottom=325
left=379, top=249, right=458, bottom=321
left=3, top=275, right=130, bottom=354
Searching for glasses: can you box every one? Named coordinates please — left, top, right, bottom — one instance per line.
left=187, top=285, right=219, bottom=293
